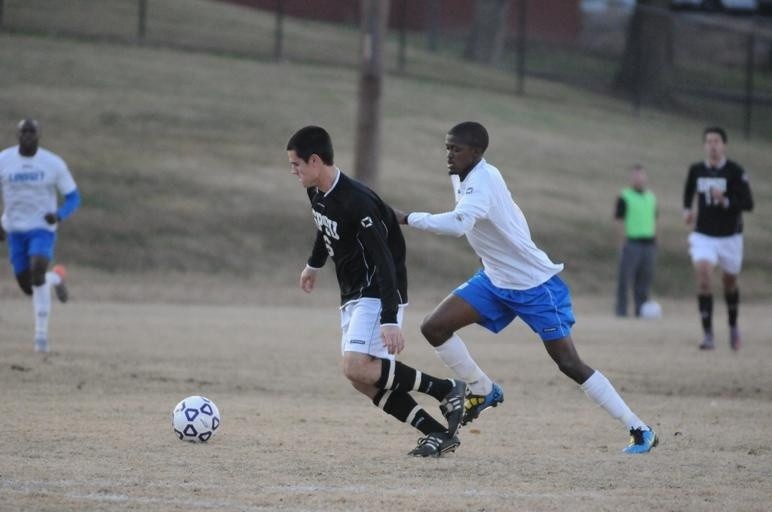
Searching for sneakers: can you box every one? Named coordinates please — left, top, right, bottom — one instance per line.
left=700, top=335, right=715, bottom=349
left=407, top=432, right=460, bottom=458
left=439, top=377, right=468, bottom=440
left=35, top=339, right=49, bottom=354
left=461, top=382, right=503, bottom=426
left=730, top=326, right=741, bottom=349
left=53, top=264, right=68, bottom=303
left=623, top=425, right=659, bottom=455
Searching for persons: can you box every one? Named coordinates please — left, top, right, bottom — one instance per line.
left=683, top=127, right=756, bottom=352
left=389, top=122, right=660, bottom=454
left=0, top=117, right=81, bottom=354
left=286, top=126, right=468, bottom=459
left=615, top=163, right=658, bottom=319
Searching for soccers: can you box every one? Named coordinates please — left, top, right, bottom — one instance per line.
left=641, top=300, right=661, bottom=320
left=171, top=396, right=220, bottom=443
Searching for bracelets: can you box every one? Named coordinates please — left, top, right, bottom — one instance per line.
left=405, top=214, right=410, bottom=225
